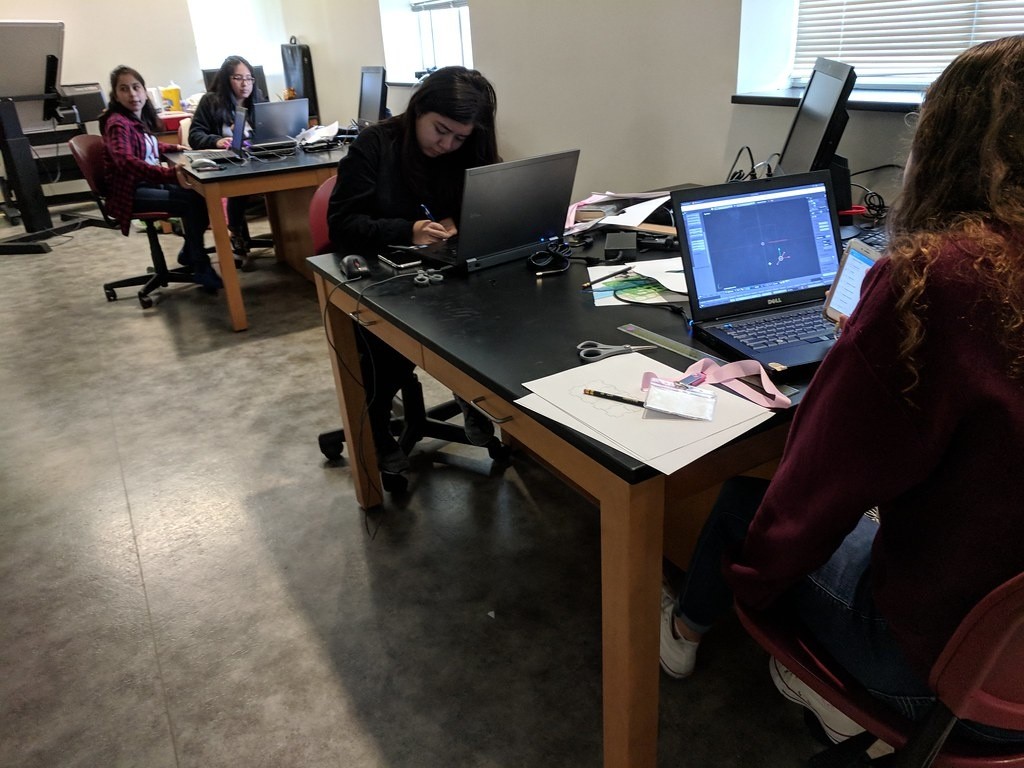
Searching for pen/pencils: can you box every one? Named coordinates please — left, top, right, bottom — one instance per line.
left=582, top=266, right=637, bottom=287
left=584, top=389, right=645, bottom=407
left=421, top=204, right=436, bottom=222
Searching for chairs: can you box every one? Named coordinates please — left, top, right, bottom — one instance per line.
left=68, top=134, right=219, bottom=308
left=731, top=570, right=1024, bottom=768
left=312, top=175, right=505, bottom=501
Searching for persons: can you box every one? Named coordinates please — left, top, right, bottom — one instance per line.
left=97, top=65, right=224, bottom=290
left=656, top=36, right=1024, bottom=745
left=188, top=56, right=269, bottom=256
left=326, top=64, right=505, bottom=475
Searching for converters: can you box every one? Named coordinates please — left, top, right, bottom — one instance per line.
left=603, top=232, right=637, bottom=260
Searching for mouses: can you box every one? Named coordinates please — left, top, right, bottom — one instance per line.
left=191, top=158, right=218, bottom=168
left=340, top=256, right=370, bottom=277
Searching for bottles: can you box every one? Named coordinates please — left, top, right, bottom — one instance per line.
left=182, top=101, right=185, bottom=112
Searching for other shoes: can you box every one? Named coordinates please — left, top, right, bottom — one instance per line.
left=368, top=407, right=402, bottom=463
left=230, top=230, right=249, bottom=254
left=177, top=249, right=224, bottom=288
left=453, top=391, right=495, bottom=446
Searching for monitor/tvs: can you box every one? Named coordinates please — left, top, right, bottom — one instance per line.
left=771, top=57, right=861, bottom=240
left=358, top=65, right=392, bottom=128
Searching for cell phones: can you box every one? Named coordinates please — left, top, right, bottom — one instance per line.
left=377, top=250, right=422, bottom=269
left=198, top=166, right=227, bottom=172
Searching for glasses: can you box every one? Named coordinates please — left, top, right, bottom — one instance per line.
left=230, top=75, right=256, bottom=85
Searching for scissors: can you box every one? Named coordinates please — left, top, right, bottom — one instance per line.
left=413, top=264, right=444, bottom=285
left=576, top=340, right=658, bottom=361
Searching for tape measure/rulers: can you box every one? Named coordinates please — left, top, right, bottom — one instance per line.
left=617, top=324, right=762, bottom=387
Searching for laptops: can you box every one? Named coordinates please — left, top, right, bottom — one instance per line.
left=670, top=170, right=848, bottom=383
left=386, top=147, right=581, bottom=273
left=249, top=97, right=311, bottom=144
left=186, top=107, right=249, bottom=163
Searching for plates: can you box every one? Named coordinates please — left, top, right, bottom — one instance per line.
left=185, top=109, right=196, bottom=113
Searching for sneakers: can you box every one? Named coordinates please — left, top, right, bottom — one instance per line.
left=657, top=584, right=698, bottom=679
left=769, top=655, right=865, bottom=745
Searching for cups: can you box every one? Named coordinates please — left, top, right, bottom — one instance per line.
left=146, top=88, right=163, bottom=113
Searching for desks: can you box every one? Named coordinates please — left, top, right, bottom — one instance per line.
left=160, top=149, right=348, bottom=332
left=308, top=182, right=797, bottom=766
